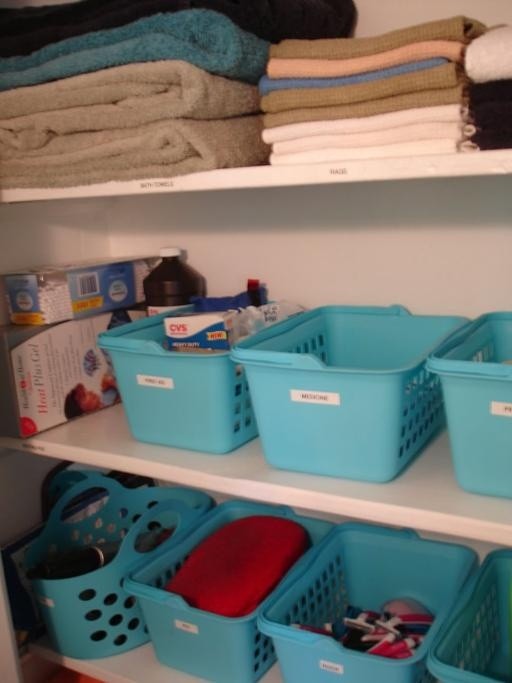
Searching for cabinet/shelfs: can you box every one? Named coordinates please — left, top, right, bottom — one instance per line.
left=0, top=148, right=510, bottom=682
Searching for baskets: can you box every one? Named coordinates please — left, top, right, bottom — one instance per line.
left=96, top=301, right=280, bottom=456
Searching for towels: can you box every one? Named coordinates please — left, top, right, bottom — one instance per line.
left=269, top=39, right=465, bottom=70
left=2, top=61, right=257, bottom=119
left=3, top=109, right=267, bottom=185
left=246, top=103, right=477, bottom=169
left=2, top=8, right=266, bottom=79
left=239, top=60, right=470, bottom=129
left=250, top=14, right=485, bottom=56
left=262, top=57, right=456, bottom=92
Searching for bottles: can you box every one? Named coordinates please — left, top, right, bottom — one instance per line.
left=143, top=247, right=199, bottom=317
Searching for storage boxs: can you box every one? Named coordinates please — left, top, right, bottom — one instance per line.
left=96, top=293, right=303, bottom=455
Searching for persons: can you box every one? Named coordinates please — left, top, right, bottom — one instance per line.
left=64, top=371, right=118, bottom=419
